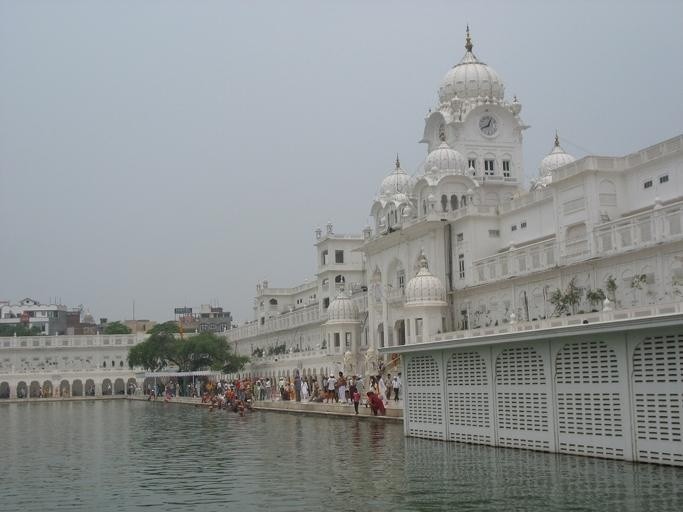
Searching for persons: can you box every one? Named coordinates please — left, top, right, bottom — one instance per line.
left=370, top=360, right=401, bottom=405
left=278, top=371, right=365, bottom=406
left=18, top=386, right=25, bottom=398
left=148, top=381, right=197, bottom=401
left=108, top=385, right=111, bottom=393
left=353, top=389, right=388, bottom=414
left=200, top=376, right=271, bottom=413
left=88, top=385, right=95, bottom=395
left=129, top=383, right=136, bottom=394
left=39, top=386, right=66, bottom=398
